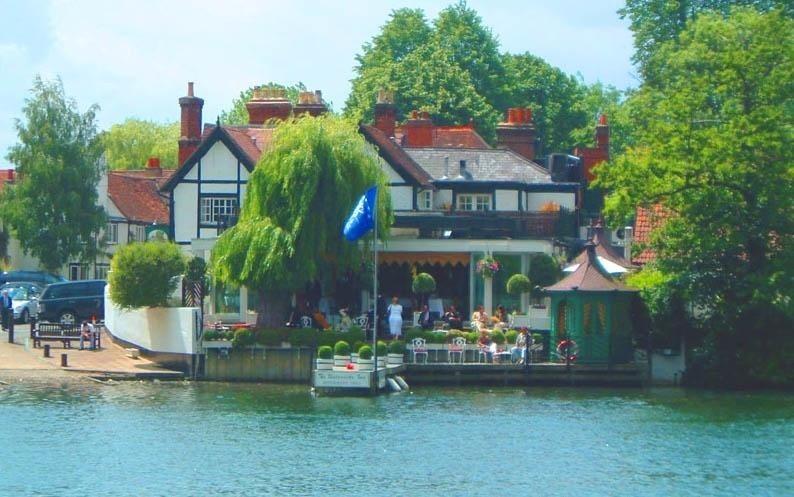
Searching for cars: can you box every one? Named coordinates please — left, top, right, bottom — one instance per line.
left=1, top=282, right=43, bottom=324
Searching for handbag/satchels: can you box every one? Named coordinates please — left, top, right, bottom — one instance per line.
left=384, top=310, right=390, bottom=318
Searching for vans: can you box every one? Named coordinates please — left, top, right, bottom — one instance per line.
left=0, top=271, right=69, bottom=287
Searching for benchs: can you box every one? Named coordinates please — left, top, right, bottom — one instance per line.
left=31, top=323, right=100, bottom=350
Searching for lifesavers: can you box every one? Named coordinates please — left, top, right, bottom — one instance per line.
left=557, top=340, right=579, bottom=362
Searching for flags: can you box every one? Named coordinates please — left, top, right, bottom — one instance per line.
left=342, top=186, right=376, bottom=242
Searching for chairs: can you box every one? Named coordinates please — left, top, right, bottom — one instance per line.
left=300, top=316, right=312, bottom=328
left=410, top=337, right=428, bottom=364
left=355, top=316, right=369, bottom=336
left=476, top=345, right=493, bottom=363
left=448, top=336, right=467, bottom=364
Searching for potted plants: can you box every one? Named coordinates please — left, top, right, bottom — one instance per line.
left=351, top=341, right=367, bottom=363
left=388, top=339, right=405, bottom=363
left=316, top=345, right=334, bottom=370
left=357, top=345, right=374, bottom=370
left=333, top=340, right=352, bottom=367
left=375, top=340, right=388, bottom=367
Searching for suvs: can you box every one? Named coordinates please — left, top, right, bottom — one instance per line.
left=36, top=279, right=107, bottom=330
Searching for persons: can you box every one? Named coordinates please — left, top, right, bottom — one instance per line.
left=510, top=325, right=532, bottom=365
left=337, top=307, right=353, bottom=329
left=442, top=303, right=463, bottom=330
left=493, top=304, right=510, bottom=328
left=77, top=320, right=95, bottom=350
left=418, top=304, right=437, bottom=330
left=476, top=330, right=499, bottom=360
left=385, top=295, right=405, bottom=340
left=0, top=290, right=13, bottom=329
left=474, top=304, right=489, bottom=332
